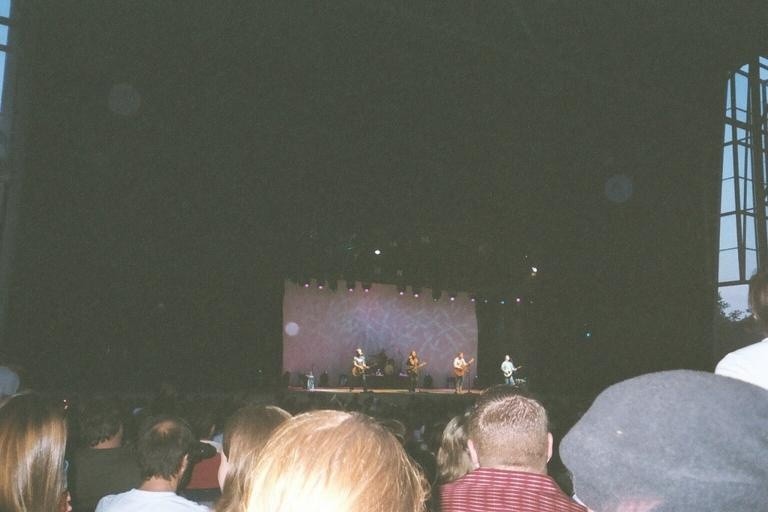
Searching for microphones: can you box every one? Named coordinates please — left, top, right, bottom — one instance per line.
left=465, top=360, right=470, bottom=365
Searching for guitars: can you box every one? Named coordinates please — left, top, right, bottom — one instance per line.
left=453, top=358, right=474, bottom=377
left=351, top=363, right=377, bottom=377
left=407, top=362, right=427, bottom=376
left=503, top=365, right=523, bottom=378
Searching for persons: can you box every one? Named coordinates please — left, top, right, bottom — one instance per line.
left=1, top=353, right=22, bottom=399
left=406, top=350, right=424, bottom=393
left=714, top=268, right=768, bottom=390
left=352, top=348, right=371, bottom=393
left=452, top=352, right=471, bottom=395
left=500, top=354, right=519, bottom=387
